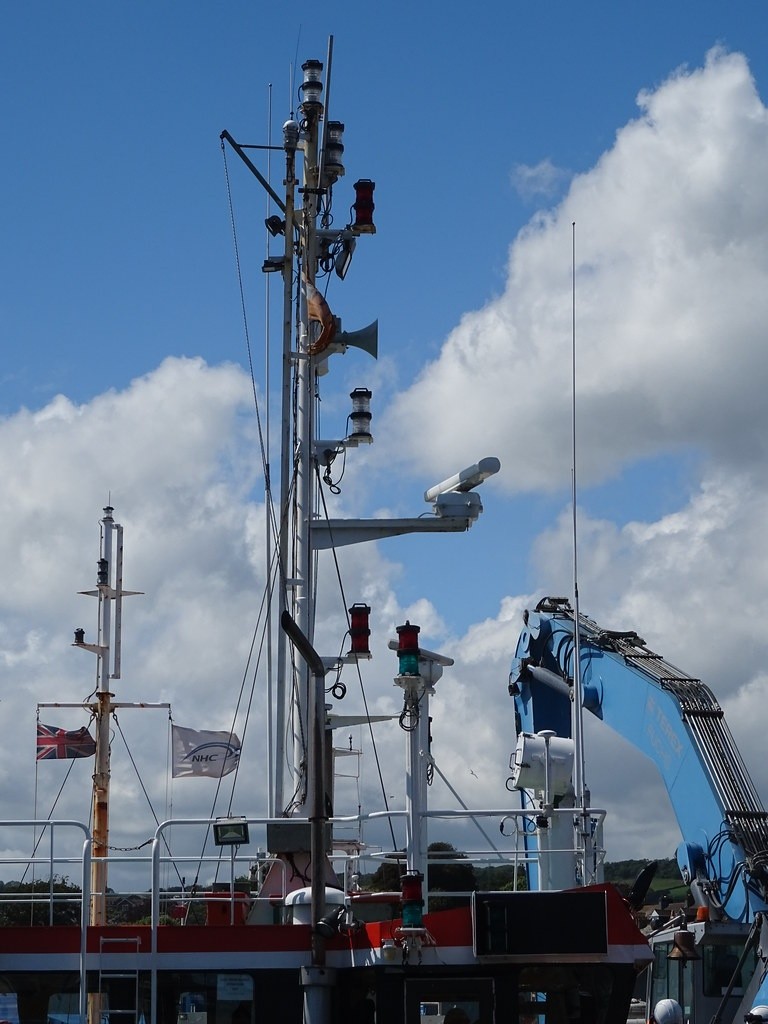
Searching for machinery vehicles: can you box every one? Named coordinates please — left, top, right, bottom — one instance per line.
left=511, top=585, right=768, bottom=1024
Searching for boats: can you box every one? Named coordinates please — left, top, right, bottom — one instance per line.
left=0, top=24, right=639, bottom=1024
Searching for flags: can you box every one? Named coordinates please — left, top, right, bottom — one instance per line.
left=36, top=719, right=98, bottom=760
left=299, top=270, right=337, bottom=355
left=169, top=722, right=241, bottom=779
